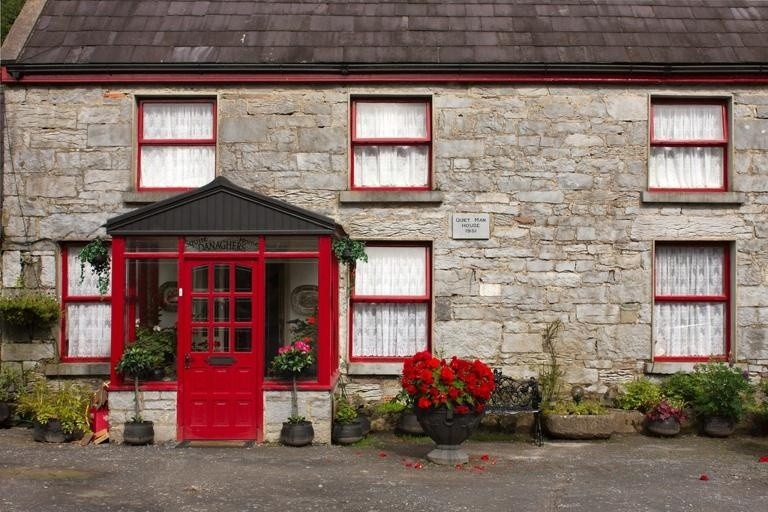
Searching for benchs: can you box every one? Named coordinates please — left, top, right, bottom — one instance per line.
left=387, top=367, right=545, bottom=446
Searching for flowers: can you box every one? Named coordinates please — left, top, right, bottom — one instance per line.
left=398, top=349, right=497, bottom=416
left=268, top=337, right=315, bottom=423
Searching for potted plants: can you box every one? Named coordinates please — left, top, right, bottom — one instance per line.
left=330, top=233, right=369, bottom=299
left=115, top=342, right=161, bottom=446
left=332, top=396, right=364, bottom=444
left=8, top=373, right=101, bottom=445
left=0, top=292, right=61, bottom=341
left=76, top=235, right=114, bottom=297
left=538, top=354, right=768, bottom=441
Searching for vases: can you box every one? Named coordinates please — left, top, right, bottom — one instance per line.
left=280, top=423, right=315, bottom=447
